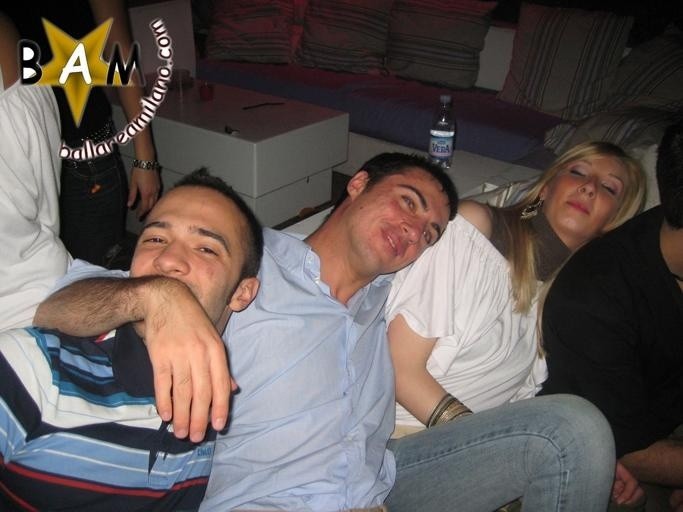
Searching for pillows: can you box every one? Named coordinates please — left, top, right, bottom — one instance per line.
left=609, top=23, right=682, bottom=112
left=384, top=0, right=493, bottom=94
left=546, top=100, right=681, bottom=156
left=204, top=3, right=293, bottom=66
left=496, top=0, right=638, bottom=119
left=294, top=1, right=392, bottom=79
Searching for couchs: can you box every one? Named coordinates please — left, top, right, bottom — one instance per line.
left=189, top=2, right=683, bottom=211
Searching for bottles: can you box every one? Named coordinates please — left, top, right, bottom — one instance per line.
left=428, top=93, right=457, bottom=169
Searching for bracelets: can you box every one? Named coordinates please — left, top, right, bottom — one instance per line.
left=422, top=391, right=471, bottom=429
left=131, top=158, right=158, bottom=171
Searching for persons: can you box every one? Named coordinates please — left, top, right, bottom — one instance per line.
left=29, top=149, right=460, bottom=511
left=1, top=169, right=264, bottom=511
left=1, top=2, right=164, bottom=269
left=538, top=119, right=683, bottom=511
left=372, top=137, right=648, bottom=511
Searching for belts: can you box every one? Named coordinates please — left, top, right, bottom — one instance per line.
left=63, top=153, right=118, bottom=171
